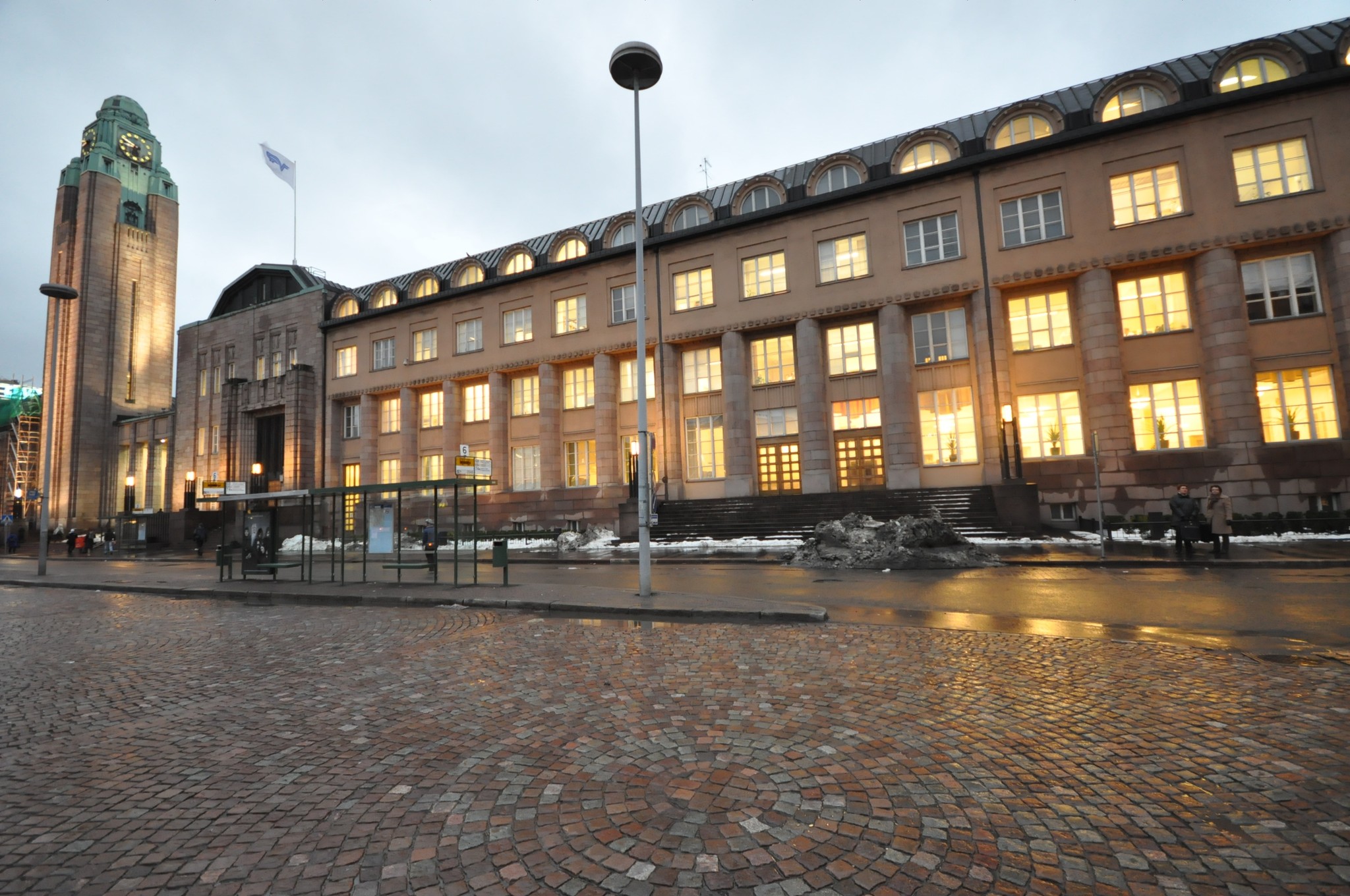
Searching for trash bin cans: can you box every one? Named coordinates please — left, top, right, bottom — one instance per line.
left=216, top=545, right=230, bottom=565
left=492, top=540, right=507, bottom=567
left=1147, top=512, right=1165, bottom=540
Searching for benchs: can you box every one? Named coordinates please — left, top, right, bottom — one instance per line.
left=256, top=563, right=302, bottom=569
left=382, top=562, right=432, bottom=570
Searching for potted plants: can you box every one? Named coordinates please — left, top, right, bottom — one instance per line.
left=947, top=431, right=958, bottom=463
left=1277, top=405, right=1300, bottom=440
left=1150, top=417, right=1170, bottom=449
left=1047, top=425, right=1061, bottom=456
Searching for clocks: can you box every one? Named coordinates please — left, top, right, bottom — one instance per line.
left=117, top=132, right=152, bottom=163
left=78, top=129, right=97, bottom=155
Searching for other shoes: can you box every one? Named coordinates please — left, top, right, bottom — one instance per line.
left=1209, top=549, right=1218, bottom=553
left=429, top=569, right=434, bottom=573
left=1187, top=553, right=1191, bottom=557
left=1174, top=549, right=1180, bottom=554
left=1221, top=550, right=1228, bottom=553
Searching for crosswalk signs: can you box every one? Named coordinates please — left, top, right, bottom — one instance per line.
left=1, top=514, right=13, bottom=525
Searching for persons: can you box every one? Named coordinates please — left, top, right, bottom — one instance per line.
left=81, top=528, right=96, bottom=556
left=193, top=523, right=207, bottom=557
left=1206, top=486, right=1232, bottom=554
left=6, top=532, right=20, bottom=553
left=243, top=523, right=270, bottom=566
left=422, top=519, right=439, bottom=573
left=1169, top=485, right=1200, bottom=556
left=104, top=529, right=116, bottom=554
left=66, top=529, right=78, bottom=557
left=18, top=525, right=26, bottom=538
left=54, top=530, right=63, bottom=543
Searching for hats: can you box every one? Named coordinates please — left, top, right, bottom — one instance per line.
left=426, top=519, right=434, bottom=524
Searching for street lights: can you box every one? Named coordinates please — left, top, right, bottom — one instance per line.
left=37, top=283, right=79, bottom=576
left=608, top=40, right=663, bottom=596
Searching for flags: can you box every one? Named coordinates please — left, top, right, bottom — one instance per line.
left=260, top=144, right=294, bottom=189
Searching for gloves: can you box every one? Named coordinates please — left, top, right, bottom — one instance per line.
left=1226, top=520, right=1232, bottom=525
